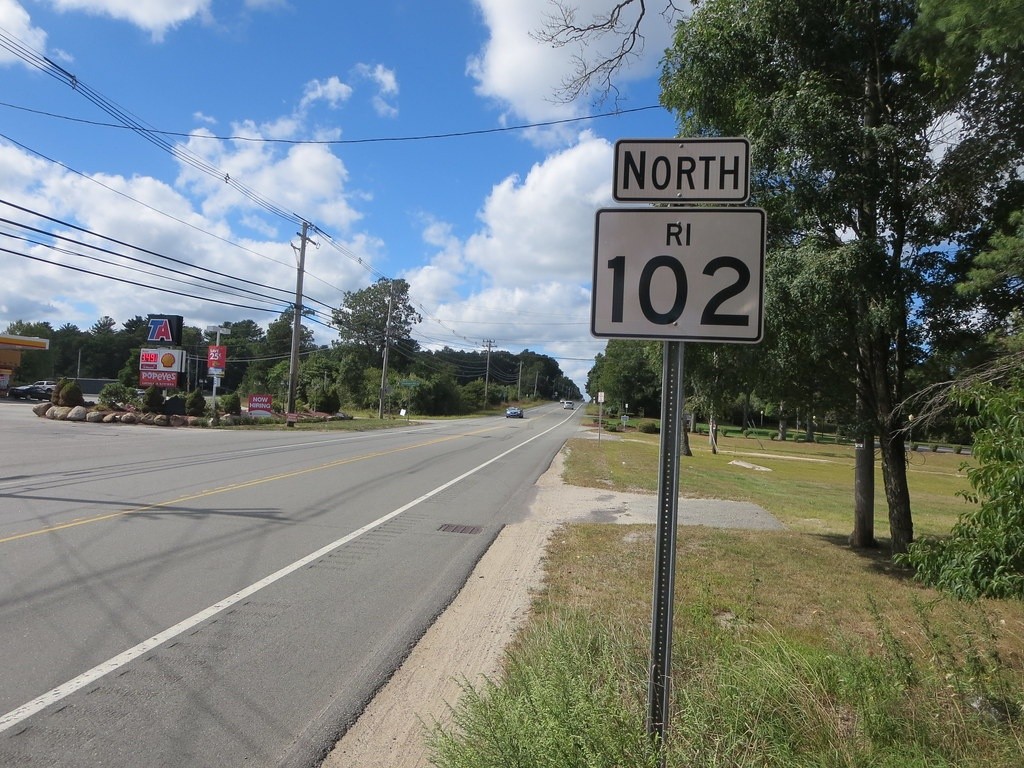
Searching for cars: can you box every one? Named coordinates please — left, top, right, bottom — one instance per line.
left=506, top=408, right=524, bottom=418
left=29, top=381, right=56, bottom=391
left=564, top=401, right=574, bottom=410
left=8, top=385, right=53, bottom=402
left=560, top=399, right=566, bottom=404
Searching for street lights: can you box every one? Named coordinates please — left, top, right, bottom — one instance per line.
left=207, top=326, right=231, bottom=410
left=761, top=411, right=763, bottom=426
left=909, top=414, right=914, bottom=451
